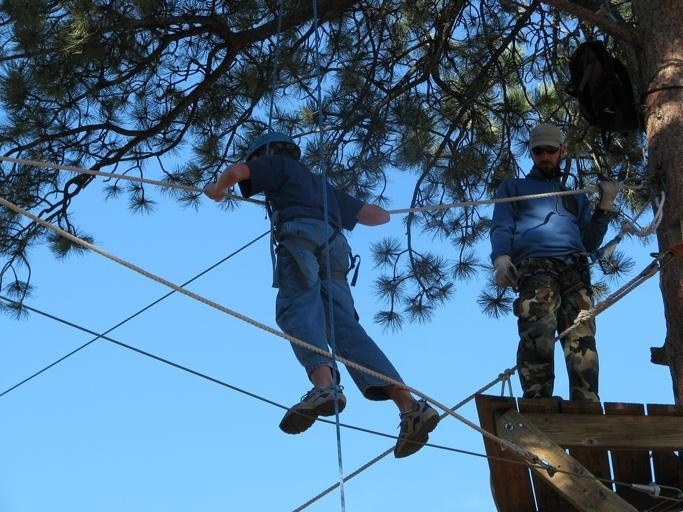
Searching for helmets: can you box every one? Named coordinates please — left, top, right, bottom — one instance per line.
left=245, top=131, right=302, bottom=162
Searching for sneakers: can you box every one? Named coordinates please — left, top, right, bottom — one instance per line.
left=280, top=382, right=346, bottom=435
left=394, top=398, right=439, bottom=460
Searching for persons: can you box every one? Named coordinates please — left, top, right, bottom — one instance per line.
left=488, top=123, right=619, bottom=400
left=202, top=131, right=438, bottom=459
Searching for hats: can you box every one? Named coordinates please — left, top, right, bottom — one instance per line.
left=527, top=122, right=565, bottom=149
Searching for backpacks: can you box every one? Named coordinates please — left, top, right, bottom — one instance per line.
left=567, top=41, right=640, bottom=130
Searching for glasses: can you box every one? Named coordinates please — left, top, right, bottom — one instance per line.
left=531, top=145, right=559, bottom=155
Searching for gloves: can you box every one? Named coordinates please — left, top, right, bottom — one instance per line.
left=596, top=179, right=619, bottom=213
left=493, top=254, right=519, bottom=287
